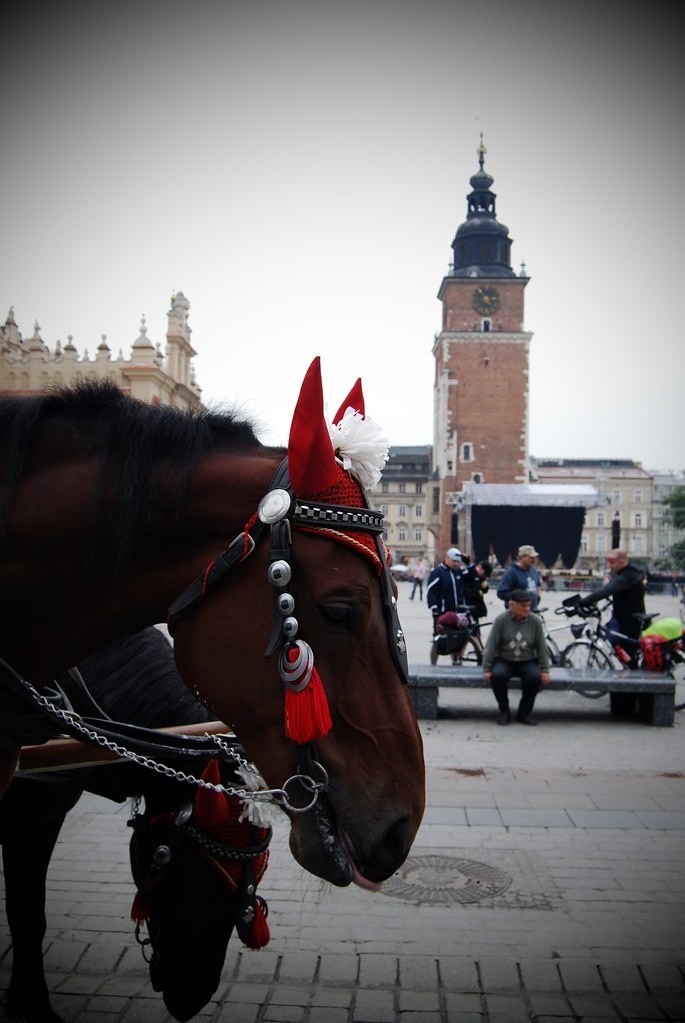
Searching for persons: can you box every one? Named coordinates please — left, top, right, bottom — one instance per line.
left=409, top=559, right=425, bottom=601
left=575, top=547, right=646, bottom=707
left=424, top=548, right=490, bottom=667
left=497, top=544, right=542, bottom=611
left=483, top=589, right=552, bottom=728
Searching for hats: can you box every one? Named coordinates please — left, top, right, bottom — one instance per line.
left=480, top=560, right=493, bottom=577
left=518, top=545, right=539, bottom=557
left=447, top=548, right=462, bottom=562
left=509, top=589, right=533, bottom=601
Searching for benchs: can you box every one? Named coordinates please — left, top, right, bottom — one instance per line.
left=405, top=664, right=676, bottom=727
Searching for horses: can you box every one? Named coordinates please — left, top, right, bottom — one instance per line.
left=0, top=357, right=428, bottom=1023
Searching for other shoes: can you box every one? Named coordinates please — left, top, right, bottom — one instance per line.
left=498, top=709, right=510, bottom=725
left=515, top=712, right=536, bottom=725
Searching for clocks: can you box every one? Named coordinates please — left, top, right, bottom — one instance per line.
left=470, top=284, right=503, bottom=316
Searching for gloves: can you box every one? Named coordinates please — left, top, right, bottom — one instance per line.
left=432, top=608, right=439, bottom=618
